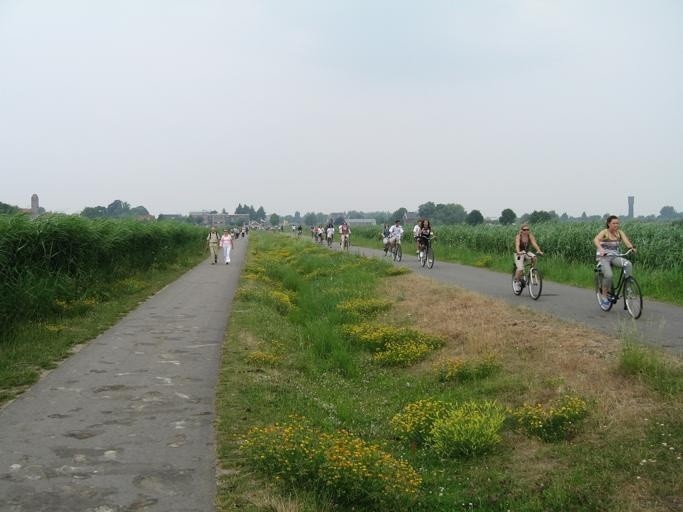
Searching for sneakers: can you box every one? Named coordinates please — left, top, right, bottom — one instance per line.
left=602, top=295, right=609, bottom=305
left=516, top=282, right=521, bottom=292
left=429, top=259, right=432, bottom=263
left=420, top=252, right=423, bottom=258
left=392, top=249, right=395, bottom=253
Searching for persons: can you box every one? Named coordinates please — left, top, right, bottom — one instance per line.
left=389, top=220, right=403, bottom=256
left=197, top=221, right=351, bottom=265
left=413, top=220, right=422, bottom=254
left=419, top=219, right=436, bottom=262
left=594, top=215, right=637, bottom=304
left=381, top=223, right=391, bottom=251
left=513, top=224, right=543, bottom=291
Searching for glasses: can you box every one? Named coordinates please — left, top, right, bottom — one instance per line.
left=522, top=229, right=529, bottom=231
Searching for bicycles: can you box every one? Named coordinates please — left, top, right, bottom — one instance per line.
left=380, top=232, right=391, bottom=257
left=594, top=246, right=645, bottom=322
left=297, top=231, right=303, bottom=240
left=310, top=231, right=335, bottom=248
left=510, top=251, right=544, bottom=300
left=339, top=234, right=351, bottom=251
left=391, top=234, right=403, bottom=263
left=414, top=234, right=437, bottom=269
